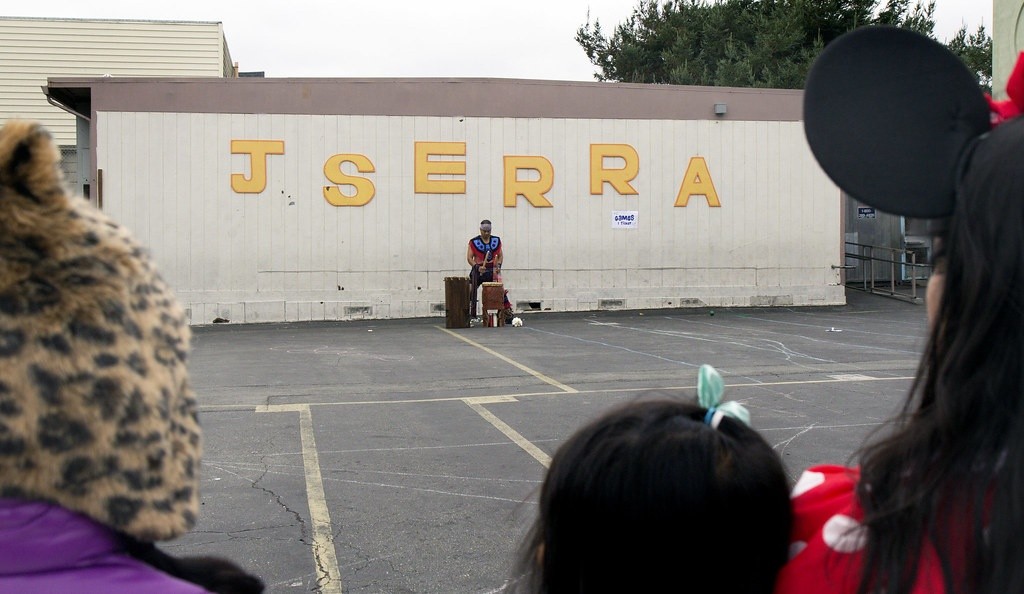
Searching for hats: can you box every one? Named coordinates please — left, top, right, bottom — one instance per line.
left=0, top=121, right=201, bottom=541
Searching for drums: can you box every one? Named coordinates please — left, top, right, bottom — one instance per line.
left=444, top=277, right=470, bottom=328
left=482, top=282, right=505, bottom=327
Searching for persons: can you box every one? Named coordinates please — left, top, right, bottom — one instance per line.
left=499, top=364, right=793, bottom=594
left=467, top=220, right=514, bottom=324
left=765, top=25, right=1024, bottom=594
left=0, top=115, right=264, bottom=594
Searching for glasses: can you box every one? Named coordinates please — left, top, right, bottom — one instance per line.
left=480, top=227, right=492, bottom=232
